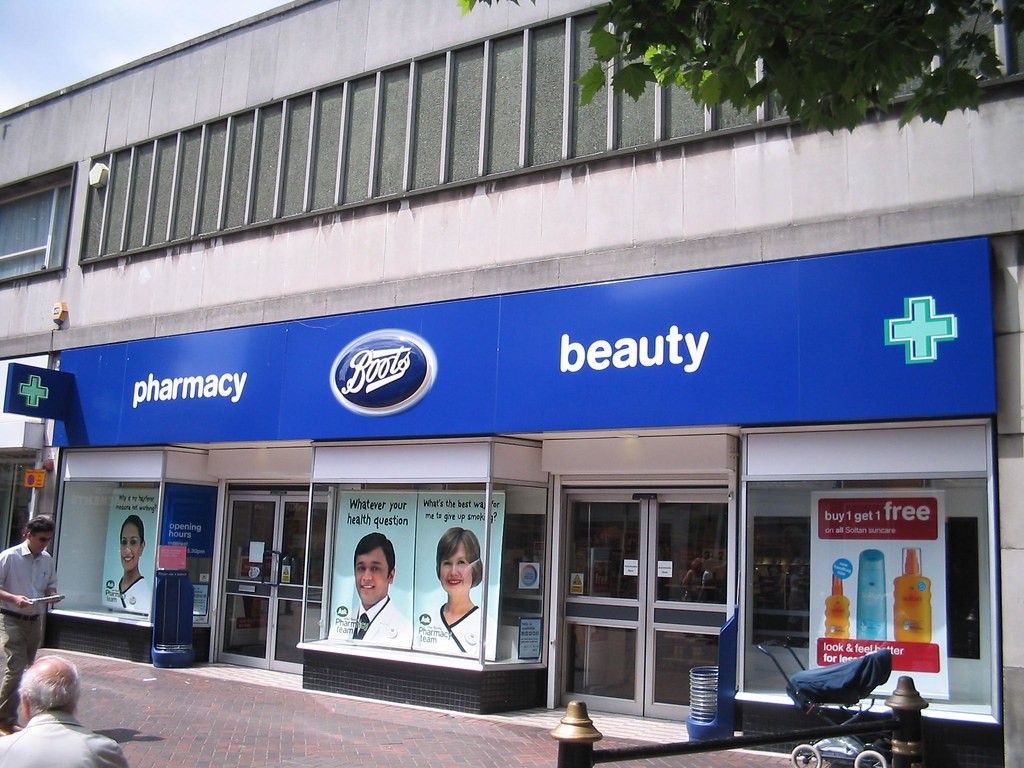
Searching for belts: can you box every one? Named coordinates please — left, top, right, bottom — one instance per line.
left=0, top=608, right=41, bottom=621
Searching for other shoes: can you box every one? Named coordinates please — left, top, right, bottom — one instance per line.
left=7, top=724, right=24, bottom=732
left=0, top=725, right=13, bottom=735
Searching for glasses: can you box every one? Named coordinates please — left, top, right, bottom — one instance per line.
left=26, top=531, right=53, bottom=543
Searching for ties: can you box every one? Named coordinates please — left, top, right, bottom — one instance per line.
left=356, top=613, right=369, bottom=639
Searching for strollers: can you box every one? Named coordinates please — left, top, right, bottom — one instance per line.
left=752, top=634, right=893, bottom=768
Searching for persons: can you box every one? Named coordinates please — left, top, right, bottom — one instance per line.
left=418, top=527, right=497, bottom=658
left=0, top=656, right=130, bottom=768
left=562, top=537, right=590, bottom=680
left=681, top=551, right=726, bottom=602
left=328, top=532, right=414, bottom=647
left=0, top=516, right=62, bottom=732
left=105, top=515, right=152, bottom=610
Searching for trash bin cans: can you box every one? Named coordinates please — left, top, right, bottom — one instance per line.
left=685, top=663, right=720, bottom=740
